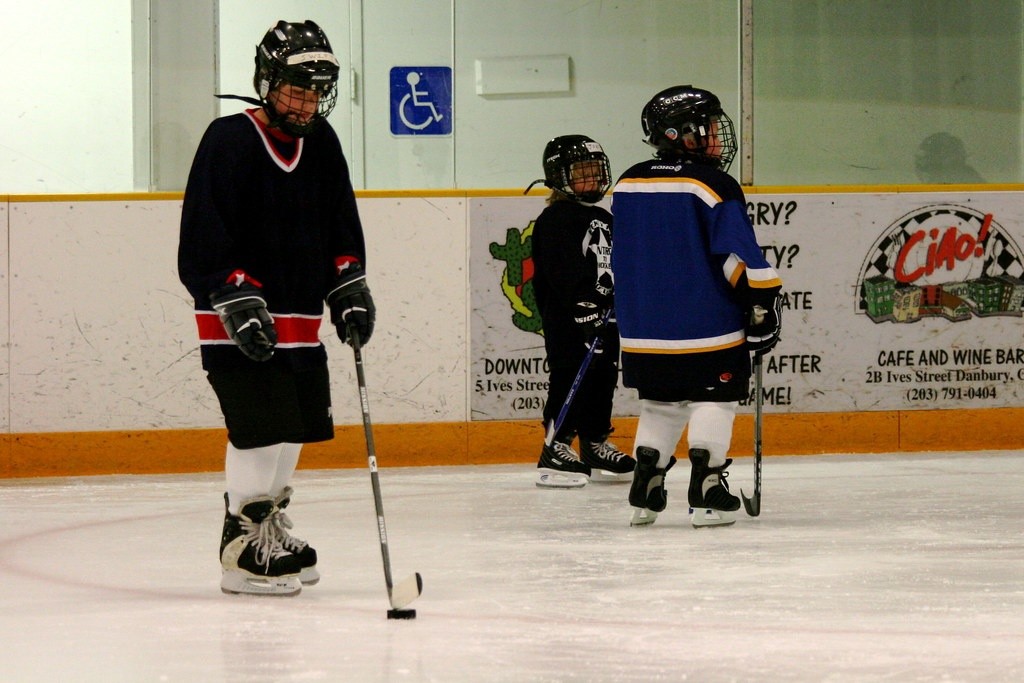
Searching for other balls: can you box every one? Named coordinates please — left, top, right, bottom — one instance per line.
left=386, top=609, right=416, bottom=619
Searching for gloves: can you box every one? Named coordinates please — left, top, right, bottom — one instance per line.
left=745, top=284, right=784, bottom=357
left=209, top=270, right=278, bottom=362
left=321, top=255, right=376, bottom=351
left=572, top=306, right=608, bottom=354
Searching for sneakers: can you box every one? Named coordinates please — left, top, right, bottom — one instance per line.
left=628, top=446, right=677, bottom=527
left=535, top=421, right=591, bottom=488
left=688, top=448, right=741, bottom=528
left=218, top=491, right=302, bottom=597
left=578, top=427, right=637, bottom=482
left=272, top=486, right=321, bottom=582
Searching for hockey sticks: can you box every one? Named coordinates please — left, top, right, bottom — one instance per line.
left=544, top=308, right=613, bottom=448
left=349, top=321, right=423, bottom=609
left=740, top=305, right=768, bottom=518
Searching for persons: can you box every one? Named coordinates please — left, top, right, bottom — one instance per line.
left=916, top=134, right=988, bottom=184
left=532, top=134, right=636, bottom=488
left=178, top=20, right=375, bottom=597
left=611, top=85, right=782, bottom=528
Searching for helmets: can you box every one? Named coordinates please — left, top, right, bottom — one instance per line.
left=543, top=135, right=612, bottom=203
left=255, top=19, right=340, bottom=138
left=641, top=85, right=738, bottom=174
